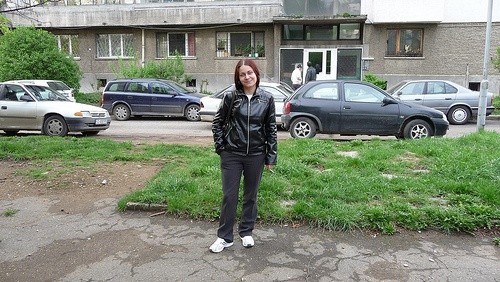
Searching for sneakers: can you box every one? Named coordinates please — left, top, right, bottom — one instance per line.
left=209, top=237, right=234, bottom=252
left=241, top=235, right=254, bottom=248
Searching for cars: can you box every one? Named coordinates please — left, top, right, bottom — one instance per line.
left=280, top=79, right=450, bottom=141
left=100, top=77, right=212, bottom=121
left=198, top=82, right=296, bottom=128
left=383, top=78, right=495, bottom=125
left=0, top=82, right=112, bottom=137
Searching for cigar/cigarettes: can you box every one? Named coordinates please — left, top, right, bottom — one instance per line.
left=269, top=169, right=275, bottom=173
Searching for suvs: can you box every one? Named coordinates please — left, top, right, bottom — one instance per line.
left=0, top=79, right=77, bottom=103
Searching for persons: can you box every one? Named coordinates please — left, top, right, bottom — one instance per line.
left=291, top=64, right=302, bottom=91
left=305, top=61, right=316, bottom=83
left=209, top=59, right=278, bottom=253
